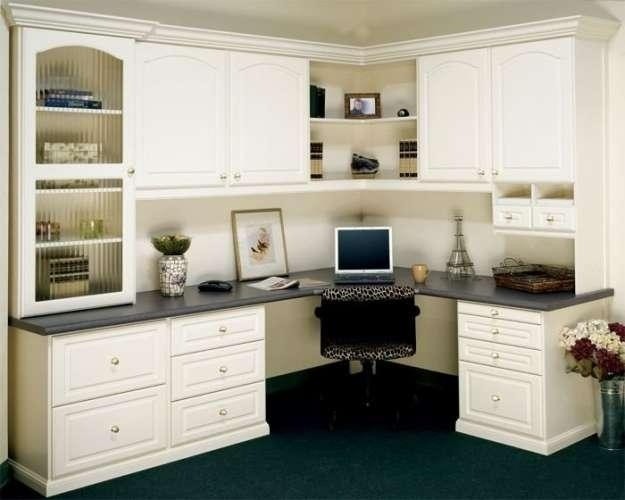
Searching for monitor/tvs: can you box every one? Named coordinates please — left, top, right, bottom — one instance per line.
left=334, top=227, right=395, bottom=274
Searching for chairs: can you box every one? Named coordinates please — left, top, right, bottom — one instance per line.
left=316, top=285, right=420, bottom=433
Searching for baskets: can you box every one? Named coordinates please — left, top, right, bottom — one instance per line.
left=493, top=265, right=575, bottom=294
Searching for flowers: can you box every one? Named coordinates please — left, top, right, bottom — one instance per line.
left=150, top=233, right=192, bottom=255
left=558, top=319, right=625, bottom=382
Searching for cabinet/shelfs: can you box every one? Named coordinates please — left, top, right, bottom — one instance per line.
left=309, top=58, right=417, bottom=192
left=9, top=26, right=136, bottom=319
left=492, top=183, right=604, bottom=238
left=416, top=37, right=606, bottom=191
left=135, top=41, right=310, bottom=199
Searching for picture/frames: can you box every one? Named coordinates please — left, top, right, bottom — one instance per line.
left=344, top=93, right=381, bottom=120
left=231, top=207, right=290, bottom=281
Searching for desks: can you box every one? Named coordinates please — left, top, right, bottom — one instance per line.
left=9, top=265, right=615, bottom=499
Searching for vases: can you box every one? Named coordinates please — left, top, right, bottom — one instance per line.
left=158, top=255, right=188, bottom=297
left=598, top=383, right=625, bottom=452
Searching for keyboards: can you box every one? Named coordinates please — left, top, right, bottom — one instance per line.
left=333, top=275, right=395, bottom=284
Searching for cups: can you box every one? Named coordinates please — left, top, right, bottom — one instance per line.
left=411, top=263, right=430, bottom=285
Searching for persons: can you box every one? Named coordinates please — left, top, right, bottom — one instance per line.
left=351, top=99, right=367, bottom=114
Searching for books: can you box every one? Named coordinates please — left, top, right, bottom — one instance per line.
left=41, top=86, right=102, bottom=109
left=310, top=84, right=326, bottom=118
left=37, top=255, right=90, bottom=300
left=40, top=141, right=103, bottom=189
left=310, top=142, right=324, bottom=178
left=247, top=276, right=332, bottom=290
left=399, top=139, right=418, bottom=178
